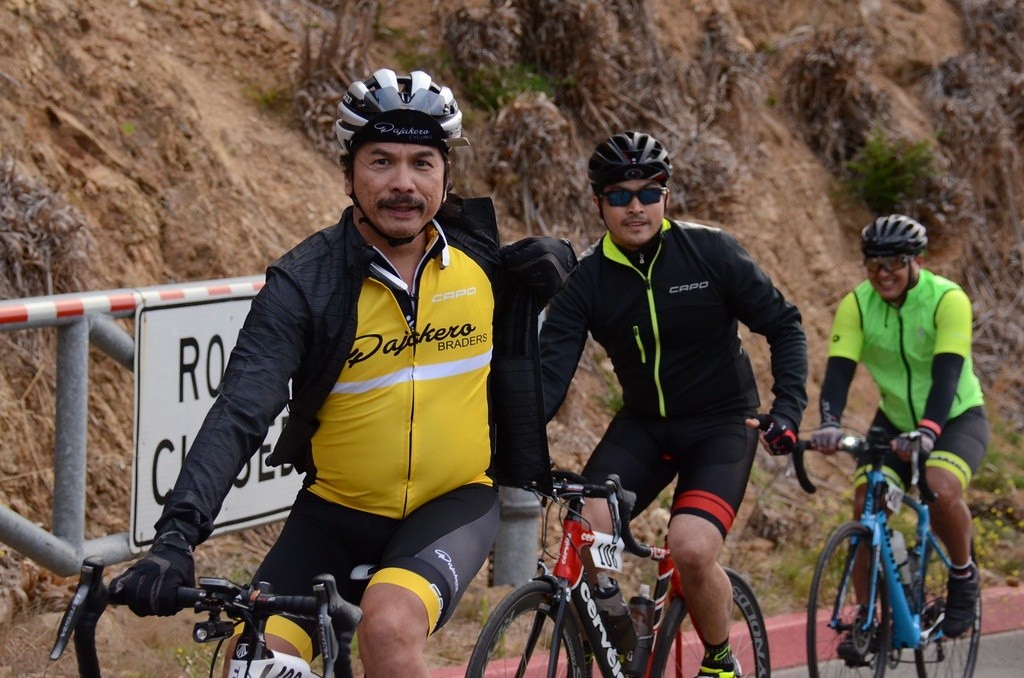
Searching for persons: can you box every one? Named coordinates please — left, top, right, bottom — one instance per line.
left=108, top=68, right=580, bottom=678
left=540, top=127, right=808, bottom=678
left=811, top=215, right=989, bottom=668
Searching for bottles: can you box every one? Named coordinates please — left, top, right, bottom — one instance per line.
left=592, top=572, right=638, bottom=655
left=619, top=584, right=655, bottom=678
left=885, top=527, right=913, bottom=585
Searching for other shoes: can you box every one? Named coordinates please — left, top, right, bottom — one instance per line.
left=693, top=656, right=742, bottom=678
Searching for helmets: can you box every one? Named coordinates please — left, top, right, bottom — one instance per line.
left=588, top=131, right=673, bottom=194
left=337, top=65, right=470, bottom=155
left=861, top=213, right=928, bottom=257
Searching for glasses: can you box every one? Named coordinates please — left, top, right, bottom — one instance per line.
left=865, top=256, right=912, bottom=271
left=602, top=188, right=667, bottom=208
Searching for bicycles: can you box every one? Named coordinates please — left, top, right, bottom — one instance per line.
left=790, top=431, right=983, bottom=678
left=463, top=457, right=772, bottom=678
left=47, top=554, right=378, bottom=678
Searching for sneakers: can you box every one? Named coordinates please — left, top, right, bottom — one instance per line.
left=838, top=634, right=865, bottom=665
left=943, top=562, right=980, bottom=637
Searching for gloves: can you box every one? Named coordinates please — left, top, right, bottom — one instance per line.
left=108, top=530, right=197, bottom=616
